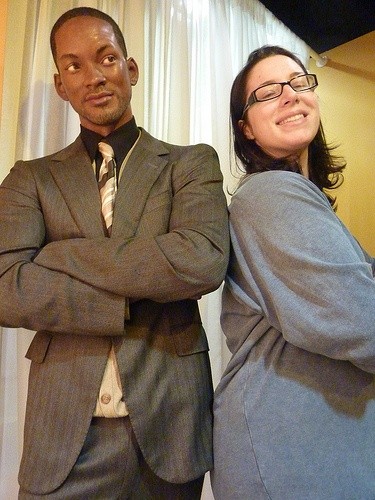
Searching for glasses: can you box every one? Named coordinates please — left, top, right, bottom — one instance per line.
left=240, top=74, right=318, bottom=120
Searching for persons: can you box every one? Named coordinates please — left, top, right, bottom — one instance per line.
left=1, top=7, right=230, bottom=500
left=208, top=44, right=374, bottom=499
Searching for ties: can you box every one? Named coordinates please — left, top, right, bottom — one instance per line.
left=98, top=142, right=117, bottom=239
